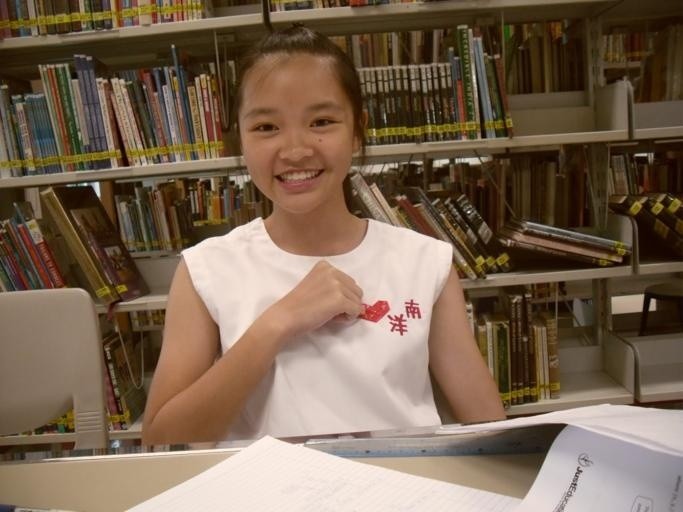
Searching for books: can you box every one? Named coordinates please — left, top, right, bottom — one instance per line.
left=114, top=176, right=274, bottom=254
left=1, top=186, right=152, bottom=307
left=270, top=1, right=400, bottom=12
left=326, top=19, right=588, bottom=147
left=98, top=309, right=167, bottom=329
left=345, top=143, right=683, bottom=279
left=463, top=285, right=561, bottom=411
left=1, top=42, right=238, bottom=178
left=603, top=20, right=683, bottom=104
left=0, top=332, right=183, bottom=460
left=1, top=1, right=215, bottom=37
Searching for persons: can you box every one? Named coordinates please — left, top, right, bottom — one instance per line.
left=143, top=22, right=506, bottom=451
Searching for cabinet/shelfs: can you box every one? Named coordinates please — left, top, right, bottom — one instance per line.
left=2, top=1, right=683, bottom=451
